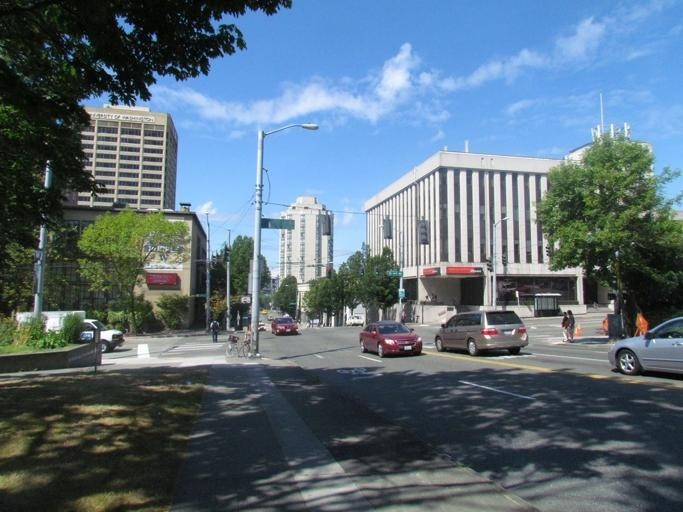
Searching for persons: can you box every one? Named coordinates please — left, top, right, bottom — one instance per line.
left=209, top=319, right=220, bottom=343
left=560, top=312, right=569, bottom=342
left=567, top=310, right=574, bottom=342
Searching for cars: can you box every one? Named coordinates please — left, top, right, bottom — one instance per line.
left=268, top=316, right=274, bottom=320
left=499, top=283, right=515, bottom=296
left=608, top=316, right=683, bottom=377
left=359, top=319, right=424, bottom=358
left=258, top=319, right=265, bottom=332
left=260, top=309, right=269, bottom=314
left=271, top=317, right=299, bottom=335
left=344, top=315, right=364, bottom=327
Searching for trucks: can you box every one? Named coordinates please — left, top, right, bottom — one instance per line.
left=14, top=310, right=126, bottom=353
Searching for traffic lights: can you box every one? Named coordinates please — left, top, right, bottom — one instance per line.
left=417, top=219, right=430, bottom=245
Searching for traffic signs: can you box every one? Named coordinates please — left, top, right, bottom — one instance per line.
left=259, top=218, right=295, bottom=230
left=387, top=271, right=403, bottom=277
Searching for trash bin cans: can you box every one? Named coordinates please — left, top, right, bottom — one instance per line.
left=608, top=314, right=622, bottom=343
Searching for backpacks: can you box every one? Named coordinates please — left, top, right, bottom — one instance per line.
left=210, top=321, right=219, bottom=334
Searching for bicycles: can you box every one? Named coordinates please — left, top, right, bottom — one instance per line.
left=224, top=335, right=252, bottom=360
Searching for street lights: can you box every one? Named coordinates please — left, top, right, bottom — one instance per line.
left=491, top=215, right=510, bottom=311
left=249, top=121, right=319, bottom=356
left=283, top=283, right=302, bottom=322
left=377, top=225, right=403, bottom=323
left=202, top=210, right=213, bottom=333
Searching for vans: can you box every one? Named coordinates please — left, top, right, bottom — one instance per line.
left=434, top=310, right=530, bottom=357
